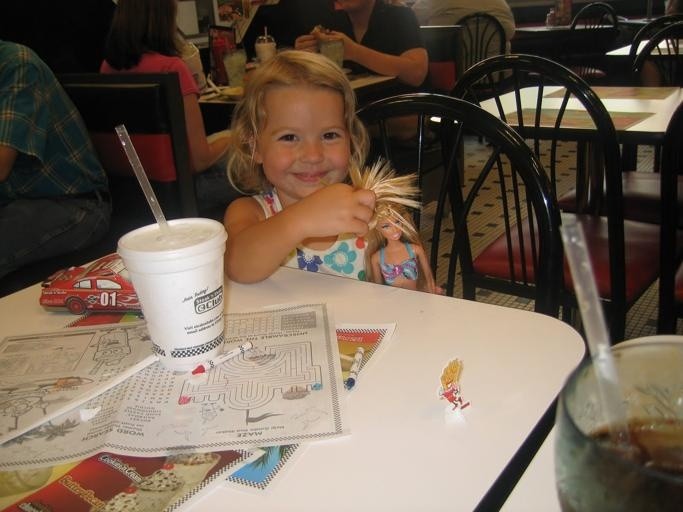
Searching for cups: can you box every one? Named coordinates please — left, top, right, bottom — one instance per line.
left=116, top=217, right=229, bottom=370
left=552, top=333, right=683, bottom=510
left=177, top=41, right=209, bottom=91
left=253, top=35, right=276, bottom=62
left=222, top=48, right=246, bottom=88
left=317, top=37, right=344, bottom=70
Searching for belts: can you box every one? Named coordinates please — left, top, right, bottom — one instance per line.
left=72, top=188, right=108, bottom=200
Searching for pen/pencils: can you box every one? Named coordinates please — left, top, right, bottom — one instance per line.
left=192, top=340, right=253, bottom=375
left=346, top=346, right=365, bottom=390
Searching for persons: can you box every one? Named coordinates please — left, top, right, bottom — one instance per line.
left=408, top=0, right=516, bottom=94
left=0, top=34, right=116, bottom=290
left=92, top=1, right=263, bottom=186
left=218, top=48, right=380, bottom=282
left=290, top=1, right=430, bottom=102
left=333, top=156, right=440, bottom=296
